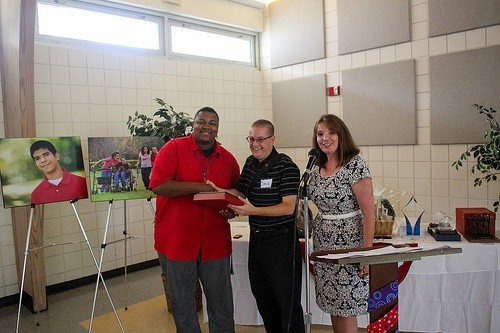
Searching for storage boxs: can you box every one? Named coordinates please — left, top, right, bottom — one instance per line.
left=455, top=207, right=497, bottom=239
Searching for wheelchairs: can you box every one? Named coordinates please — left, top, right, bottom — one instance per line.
left=110, top=166, right=137, bottom=193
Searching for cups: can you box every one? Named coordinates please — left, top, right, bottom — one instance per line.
left=471, top=214, right=490, bottom=236
left=432, top=212, right=456, bottom=232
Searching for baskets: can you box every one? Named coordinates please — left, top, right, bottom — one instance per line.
left=375, top=219, right=394, bottom=235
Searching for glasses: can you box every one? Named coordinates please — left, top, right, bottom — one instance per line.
left=245, top=136, right=271, bottom=143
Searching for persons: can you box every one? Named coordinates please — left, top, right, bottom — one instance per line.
left=136, top=146, right=158, bottom=187
left=308, top=115, right=376, bottom=333
left=91, top=152, right=131, bottom=192
left=148, top=107, right=240, bottom=333
left=30, top=140, right=88, bottom=203
left=206, top=120, right=305, bottom=333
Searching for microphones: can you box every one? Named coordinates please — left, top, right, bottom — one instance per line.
left=300, top=148, right=321, bottom=180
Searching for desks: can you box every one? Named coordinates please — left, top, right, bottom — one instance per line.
left=201, top=222, right=500, bottom=333
